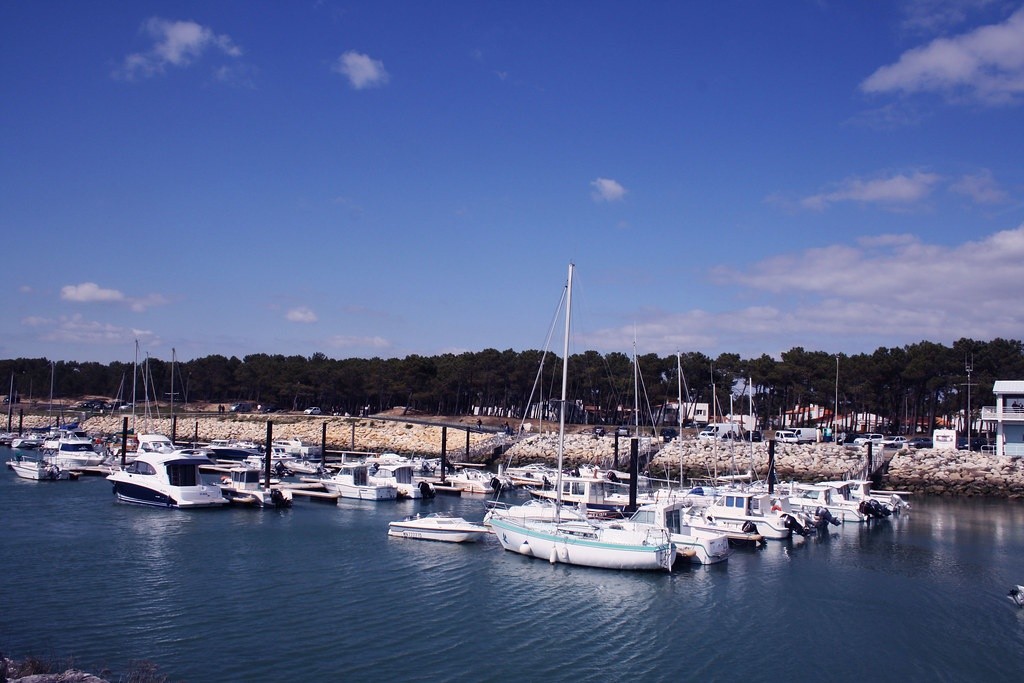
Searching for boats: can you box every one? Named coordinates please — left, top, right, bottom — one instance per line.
left=0, top=339, right=580, bottom=506
left=387, top=511, right=489, bottom=544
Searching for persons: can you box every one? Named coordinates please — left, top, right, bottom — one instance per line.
left=331, top=406, right=343, bottom=415
left=1012, top=401, right=1024, bottom=413
left=476, top=419, right=482, bottom=428
left=218, top=404, right=225, bottom=412
left=109, top=451, right=115, bottom=461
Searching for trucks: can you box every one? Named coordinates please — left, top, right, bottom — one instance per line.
left=699, top=422, right=739, bottom=441
left=786, top=428, right=821, bottom=445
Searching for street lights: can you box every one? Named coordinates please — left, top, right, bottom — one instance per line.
left=536, top=360, right=545, bottom=436
left=23, top=370, right=33, bottom=409
left=185, top=371, right=193, bottom=414
left=834, top=354, right=840, bottom=445
left=905, top=389, right=909, bottom=435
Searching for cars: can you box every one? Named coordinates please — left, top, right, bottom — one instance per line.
left=972, top=438, right=989, bottom=450
left=94, top=403, right=113, bottom=412
left=617, top=426, right=632, bottom=436
left=592, top=426, right=605, bottom=436
left=838, top=433, right=867, bottom=444
left=107, top=400, right=128, bottom=410
left=230, top=402, right=251, bottom=413
left=3, top=395, right=21, bottom=404
left=959, top=438, right=976, bottom=451
left=774, top=430, right=799, bottom=444
left=744, top=431, right=761, bottom=443
left=884, top=436, right=907, bottom=448
left=660, top=428, right=677, bottom=442
left=304, top=407, right=321, bottom=415
left=118, top=403, right=140, bottom=412
left=80, top=400, right=104, bottom=409
left=907, top=438, right=933, bottom=448
left=854, top=434, right=885, bottom=447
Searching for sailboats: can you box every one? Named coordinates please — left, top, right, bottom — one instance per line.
left=581, top=334, right=908, bottom=564
left=489, top=258, right=677, bottom=571
